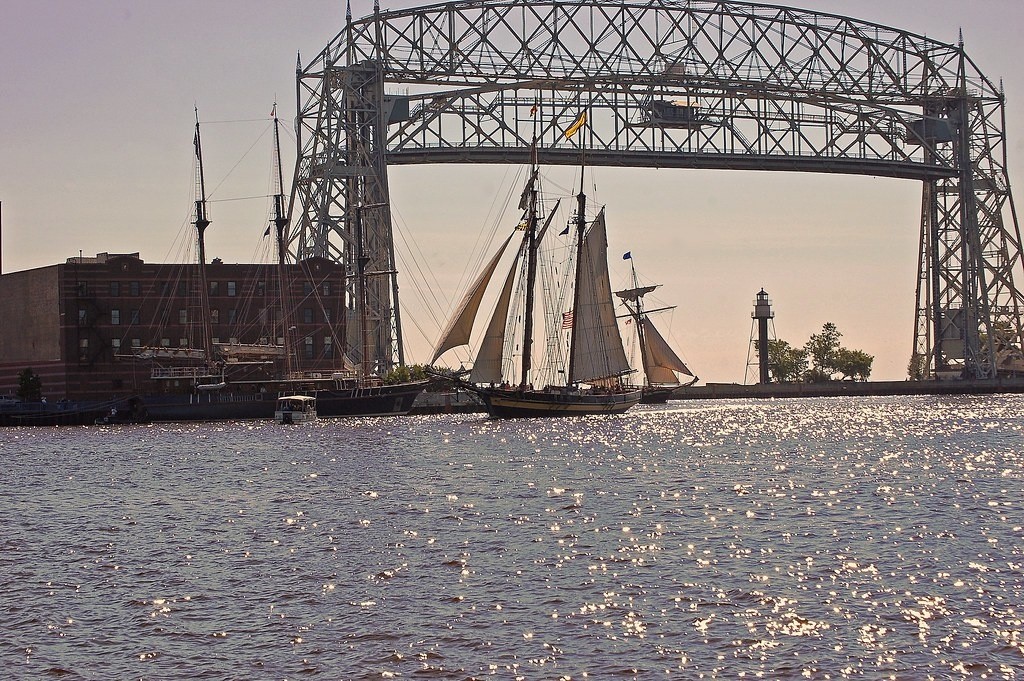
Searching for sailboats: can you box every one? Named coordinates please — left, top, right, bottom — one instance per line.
left=608, top=251, right=699, bottom=404
left=97, top=104, right=431, bottom=422
left=419, top=94, right=646, bottom=415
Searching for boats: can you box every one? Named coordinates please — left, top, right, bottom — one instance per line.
left=94, top=418, right=131, bottom=424
left=272, top=395, right=317, bottom=425
left=931, top=348, right=1024, bottom=379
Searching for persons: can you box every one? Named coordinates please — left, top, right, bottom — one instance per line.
left=586, top=379, right=634, bottom=395
left=488, top=376, right=534, bottom=393
left=637, top=383, right=662, bottom=392
left=279, top=401, right=314, bottom=413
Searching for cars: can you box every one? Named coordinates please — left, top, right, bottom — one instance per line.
left=0, top=395, right=21, bottom=406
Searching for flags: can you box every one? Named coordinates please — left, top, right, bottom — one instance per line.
left=530, top=105, right=538, bottom=117
left=562, top=310, right=573, bottom=329
left=623, top=251, right=631, bottom=260
left=565, top=110, right=587, bottom=140
left=559, top=225, right=570, bottom=237
